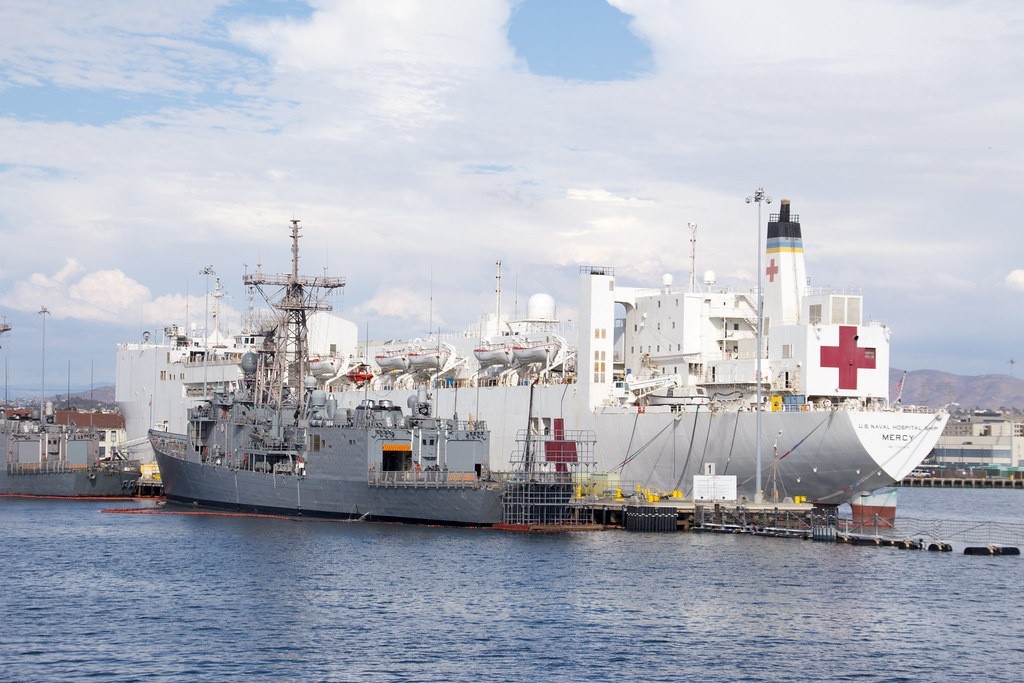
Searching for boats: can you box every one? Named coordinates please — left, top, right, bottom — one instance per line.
left=1, top=418, right=142, bottom=499
left=144, top=212, right=578, bottom=526
left=113, top=184, right=952, bottom=509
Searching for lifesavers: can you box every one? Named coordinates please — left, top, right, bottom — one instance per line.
left=89, top=473, right=96, bottom=479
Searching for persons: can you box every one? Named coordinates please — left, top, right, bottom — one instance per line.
left=294, top=406, right=301, bottom=427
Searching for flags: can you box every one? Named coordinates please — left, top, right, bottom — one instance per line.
left=0, top=323, right=12, bottom=334
